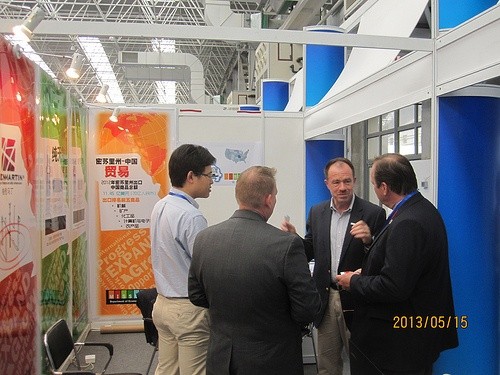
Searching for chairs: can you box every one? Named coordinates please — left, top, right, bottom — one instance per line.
left=136, top=288, right=161, bottom=375
left=43, top=319, right=113, bottom=375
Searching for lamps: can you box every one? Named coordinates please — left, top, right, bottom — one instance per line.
left=13, top=6, right=47, bottom=44
left=108, top=107, right=121, bottom=123
left=95, top=84, right=110, bottom=105
left=65, top=52, right=87, bottom=78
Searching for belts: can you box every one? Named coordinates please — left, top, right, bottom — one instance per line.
left=330, top=283, right=340, bottom=291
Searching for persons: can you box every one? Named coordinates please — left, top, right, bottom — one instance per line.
left=281, top=157, right=387, bottom=375
left=335, top=152, right=460, bottom=375
left=150, top=142, right=217, bottom=375
left=188, top=165, right=323, bottom=375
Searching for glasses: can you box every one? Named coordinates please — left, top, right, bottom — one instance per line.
left=200, top=172, right=212, bottom=178
